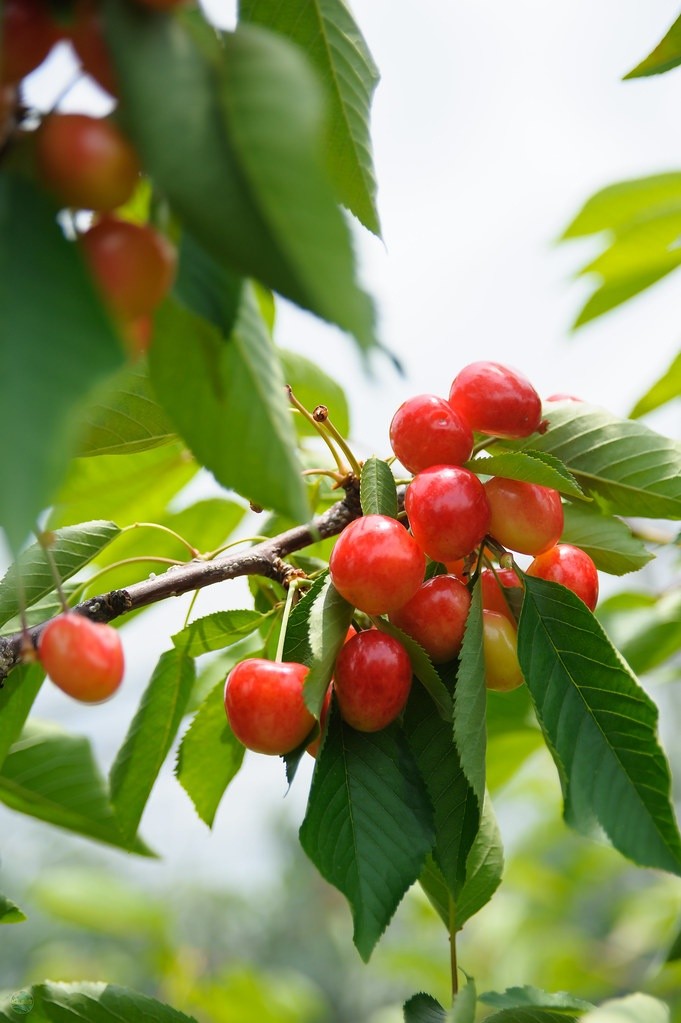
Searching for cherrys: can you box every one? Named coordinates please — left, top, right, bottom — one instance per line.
left=40, top=362, right=599, bottom=757
left=0, top=0, right=191, bottom=353
